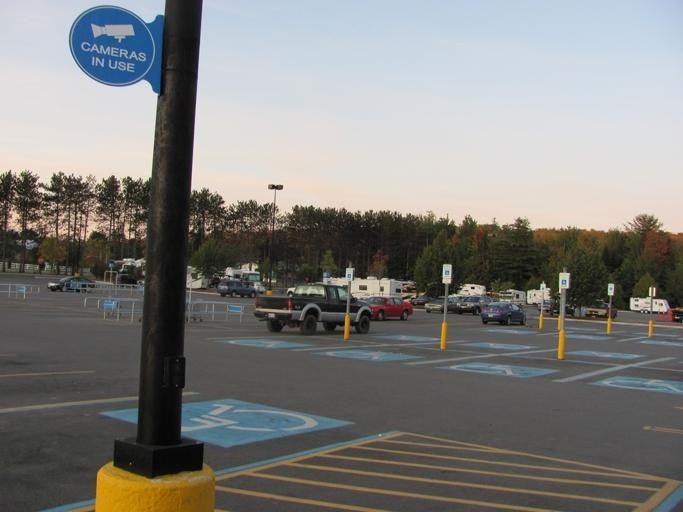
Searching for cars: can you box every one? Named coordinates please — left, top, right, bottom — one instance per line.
left=411, top=294, right=488, bottom=315
left=366, top=295, right=414, bottom=321
left=47, top=275, right=97, bottom=293
left=481, top=301, right=527, bottom=325
left=584, top=299, right=618, bottom=318
left=537, top=299, right=574, bottom=316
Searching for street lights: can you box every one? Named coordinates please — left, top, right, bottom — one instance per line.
left=266, top=184, right=284, bottom=296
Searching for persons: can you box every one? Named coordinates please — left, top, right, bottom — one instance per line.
left=37, top=255, right=43, bottom=275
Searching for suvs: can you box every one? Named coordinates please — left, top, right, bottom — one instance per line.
left=217, top=280, right=256, bottom=298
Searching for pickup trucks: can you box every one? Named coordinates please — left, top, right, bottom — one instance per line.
left=253, top=283, right=372, bottom=336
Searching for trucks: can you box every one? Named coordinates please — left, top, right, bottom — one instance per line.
left=629, top=297, right=670, bottom=314
